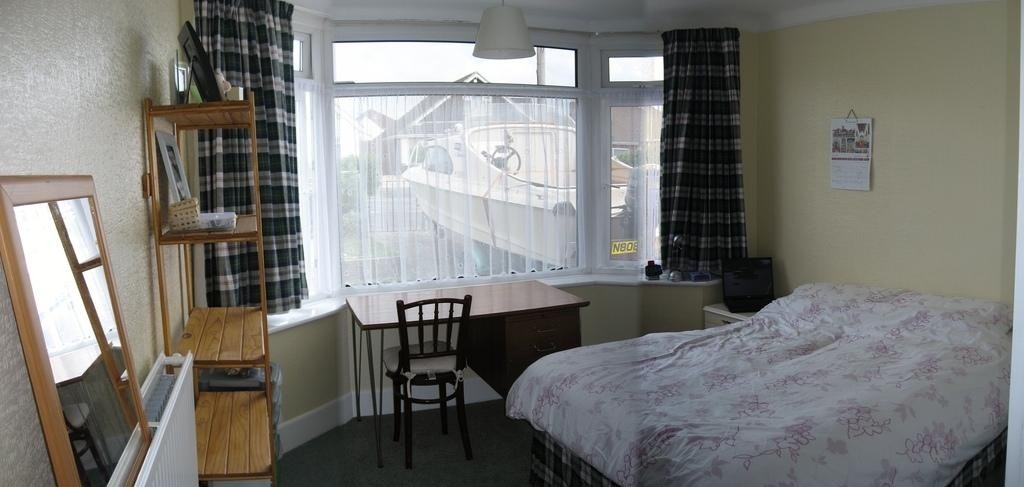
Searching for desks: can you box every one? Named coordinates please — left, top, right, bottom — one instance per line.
left=344, top=279, right=589, bottom=467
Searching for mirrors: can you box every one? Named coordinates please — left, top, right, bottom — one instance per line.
left=1, top=175, right=153, bottom=486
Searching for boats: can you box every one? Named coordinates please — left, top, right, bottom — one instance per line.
left=404, top=124, right=661, bottom=269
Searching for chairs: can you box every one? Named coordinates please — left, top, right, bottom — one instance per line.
left=382, top=295, right=475, bottom=469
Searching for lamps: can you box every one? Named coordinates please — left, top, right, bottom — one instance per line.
left=472, top=0, right=536, bottom=60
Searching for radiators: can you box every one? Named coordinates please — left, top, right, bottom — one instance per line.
left=107, top=351, right=200, bottom=487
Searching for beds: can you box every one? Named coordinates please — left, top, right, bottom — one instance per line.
left=506, top=281, right=1014, bottom=487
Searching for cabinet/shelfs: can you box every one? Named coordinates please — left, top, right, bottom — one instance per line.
left=141, top=92, right=274, bottom=487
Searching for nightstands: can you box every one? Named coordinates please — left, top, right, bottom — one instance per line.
left=703, top=301, right=756, bottom=328
